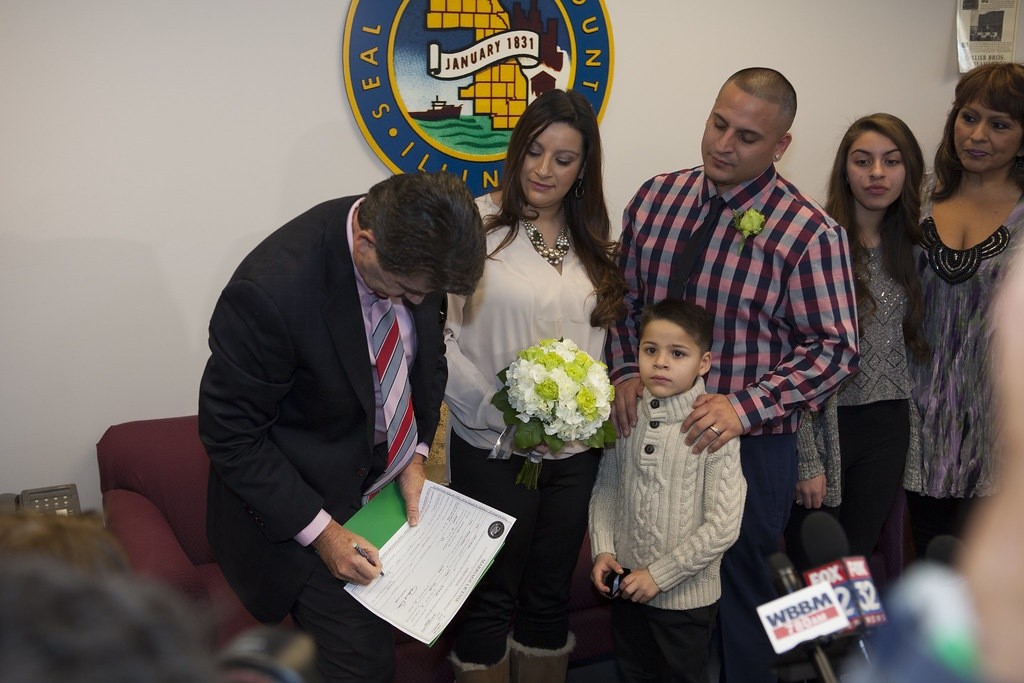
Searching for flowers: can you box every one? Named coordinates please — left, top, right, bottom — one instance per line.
left=488, top=336, right=618, bottom=492
left=731, top=206, right=765, bottom=258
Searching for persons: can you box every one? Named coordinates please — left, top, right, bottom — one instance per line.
left=584, top=61, right=1024, bottom=683
left=444, top=89, right=625, bottom=683
left=198, top=172, right=486, bottom=683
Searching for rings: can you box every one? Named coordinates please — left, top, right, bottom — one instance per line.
left=711, top=426, right=722, bottom=437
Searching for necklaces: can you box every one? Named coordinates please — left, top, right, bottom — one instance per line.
left=518, top=201, right=570, bottom=266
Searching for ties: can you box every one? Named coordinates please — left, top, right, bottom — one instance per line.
left=362, top=294, right=419, bottom=508
left=666, top=195, right=728, bottom=300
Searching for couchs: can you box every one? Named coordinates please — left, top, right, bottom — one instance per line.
left=95, top=414, right=917, bottom=683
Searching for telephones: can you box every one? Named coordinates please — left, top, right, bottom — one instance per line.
left=0, top=484, right=80, bottom=519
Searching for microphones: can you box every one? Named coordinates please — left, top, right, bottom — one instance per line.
left=745, top=551, right=850, bottom=683
left=802, top=512, right=888, bottom=664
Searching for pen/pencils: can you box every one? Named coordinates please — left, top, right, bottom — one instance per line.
left=353, top=544, right=384, bottom=576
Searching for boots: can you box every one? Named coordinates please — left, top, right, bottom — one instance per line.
left=449, top=633, right=576, bottom=683
